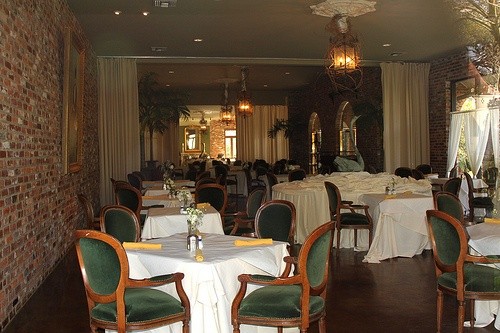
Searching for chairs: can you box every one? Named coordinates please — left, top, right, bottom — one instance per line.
left=74, top=151, right=500, bottom=333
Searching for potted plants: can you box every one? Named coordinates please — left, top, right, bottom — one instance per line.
left=138, top=71, right=196, bottom=180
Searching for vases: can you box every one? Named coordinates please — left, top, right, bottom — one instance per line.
left=187, top=231, right=198, bottom=250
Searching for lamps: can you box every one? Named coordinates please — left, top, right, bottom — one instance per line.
left=236, top=69, right=254, bottom=119
left=220, top=83, right=232, bottom=115
left=323, top=12, right=363, bottom=100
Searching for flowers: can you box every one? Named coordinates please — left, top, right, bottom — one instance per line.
left=186, top=207, right=203, bottom=233
left=163, top=178, right=176, bottom=192
left=176, top=187, right=192, bottom=206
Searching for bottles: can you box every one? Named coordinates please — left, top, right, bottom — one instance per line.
left=385, top=187, right=396, bottom=195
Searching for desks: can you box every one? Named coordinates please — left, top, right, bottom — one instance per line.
left=357, top=192, right=431, bottom=263
left=143, top=194, right=194, bottom=208
left=105, top=232, right=300, bottom=333
left=141, top=206, right=225, bottom=242
left=142, top=180, right=195, bottom=189
left=464, top=220, right=500, bottom=331
left=270, top=171, right=431, bottom=250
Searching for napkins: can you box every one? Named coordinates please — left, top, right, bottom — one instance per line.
left=484, top=218, right=500, bottom=224
left=122, top=242, right=161, bottom=250
left=233, top=238, right=273, bottom=247
left=196, top=249, right=204, bottom=261
